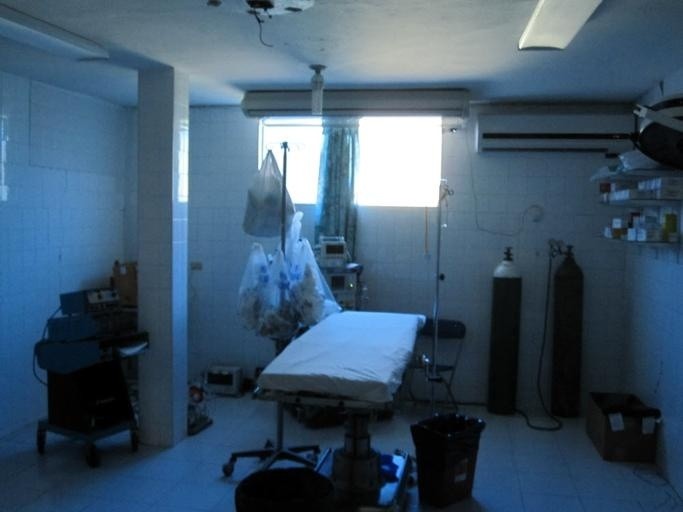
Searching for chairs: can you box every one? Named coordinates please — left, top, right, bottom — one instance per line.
left=397, top=316, right=466, bottom=412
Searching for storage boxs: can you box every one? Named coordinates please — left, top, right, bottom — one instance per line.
left=111, top=260, right=137, bottom=307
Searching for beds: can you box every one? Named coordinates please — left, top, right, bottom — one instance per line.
left=257, top=310, right=425, bottom=454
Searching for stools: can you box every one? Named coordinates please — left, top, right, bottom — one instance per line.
left=234, top=466, right=335, bottom=511
left=222, top=390, right=322, bottom=470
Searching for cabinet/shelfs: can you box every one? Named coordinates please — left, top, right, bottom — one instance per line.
left=601, top=167, right=680, bottom=249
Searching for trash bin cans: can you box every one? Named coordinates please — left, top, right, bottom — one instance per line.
left=410, top=413, right=484, bottom=508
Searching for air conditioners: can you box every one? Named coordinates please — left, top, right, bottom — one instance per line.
left=472, top=112, right=636, bottom=156
